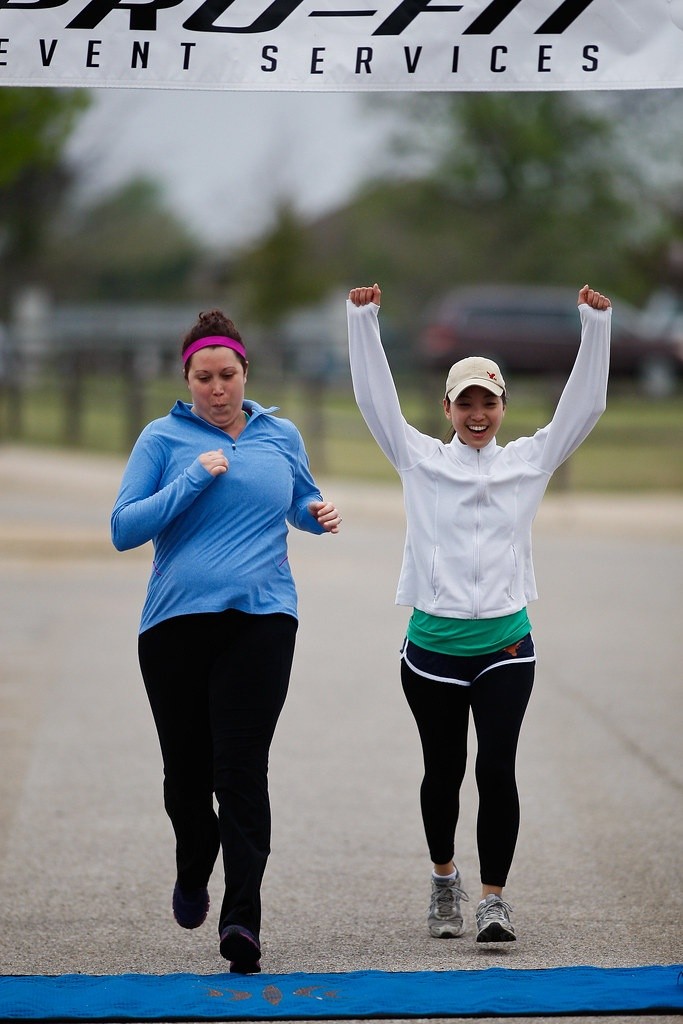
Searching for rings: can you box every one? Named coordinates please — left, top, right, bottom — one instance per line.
left=339, top=517, right=342, bottom=521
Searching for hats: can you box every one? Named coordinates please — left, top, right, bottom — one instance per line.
left=445, top=356, right=508, bottom=404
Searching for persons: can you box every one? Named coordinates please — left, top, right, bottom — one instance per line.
left=346, top=284, right=612, bottom=942
left=110, top=311, right=341, bottom=973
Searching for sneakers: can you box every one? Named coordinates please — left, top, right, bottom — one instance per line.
left=473, top=894, right=516, bottom=943
left=429, top=860, right=467, bottom=936
left=173, top=871, right=212, bottom=929
left=219, top=925, right=261, bottom=974
